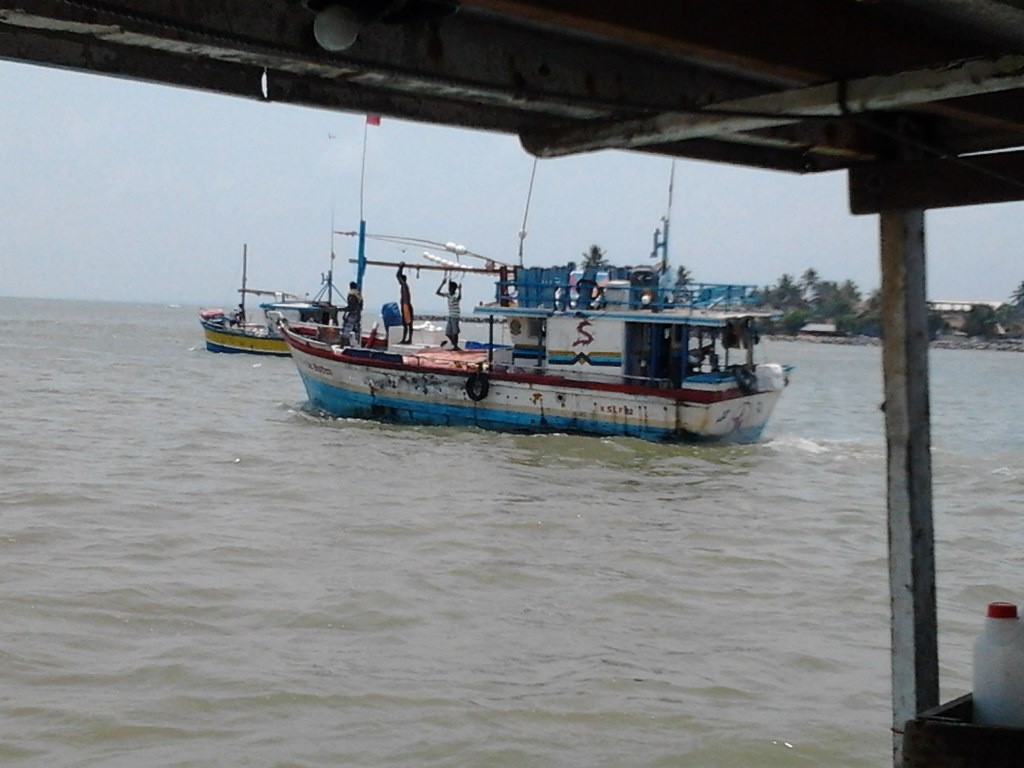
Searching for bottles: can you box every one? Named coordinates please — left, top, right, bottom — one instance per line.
left=970, top=601, right=1024, bottom=728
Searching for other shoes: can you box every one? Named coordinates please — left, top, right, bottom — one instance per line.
left=452, top=346, right=461, bottom=351
left=406, top=340, right=412, bottom=345
left=397, top=340, right=406, bottom=344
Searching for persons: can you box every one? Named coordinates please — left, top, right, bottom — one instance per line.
left=437, top=279, right=462, bottom=351
left=337, top=280, right=363, bottom=347
left=236, top=304, right=246, bottom=322
left=395, top=262, right=414, bottom=345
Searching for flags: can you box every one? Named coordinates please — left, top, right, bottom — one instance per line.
left=367, top=115, right=381, bottom=126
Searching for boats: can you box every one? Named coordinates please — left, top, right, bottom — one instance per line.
left=198, top=243, right=389, bottom=358
left=266, top=107, right=798, bottom=455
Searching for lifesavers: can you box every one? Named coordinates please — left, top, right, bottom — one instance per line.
left=465, top=375, right=489, bottom=401
left=735, top=368, right=756, bottom=393
